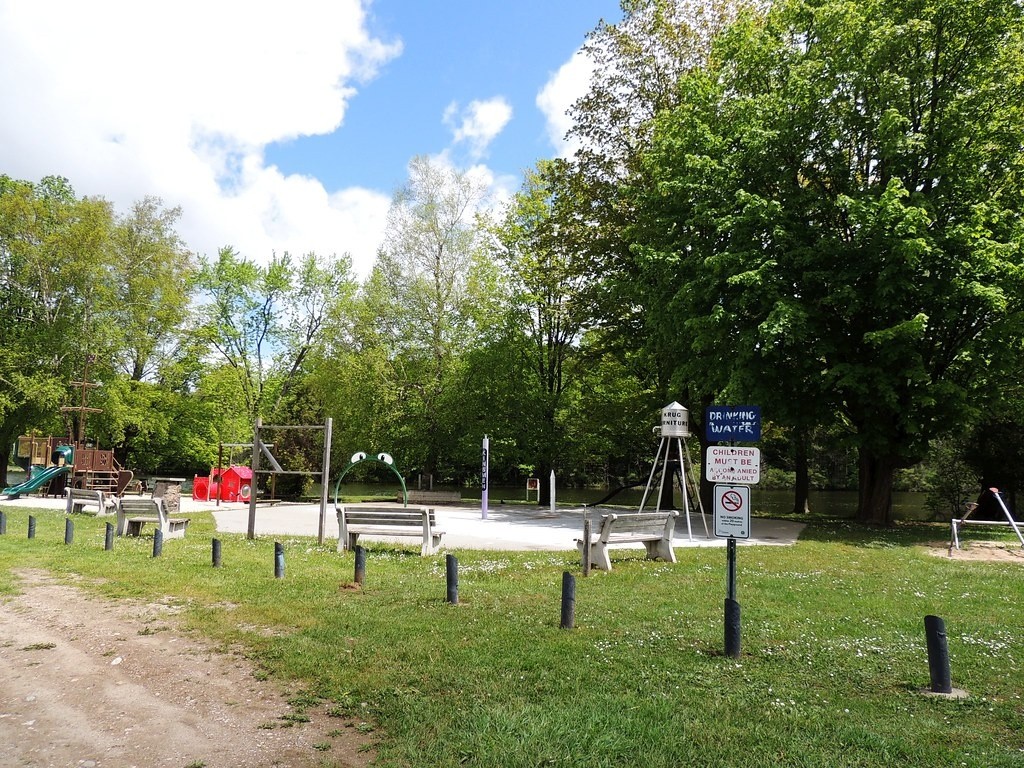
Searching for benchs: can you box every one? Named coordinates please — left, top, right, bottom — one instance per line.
left=111, top=498, right=191, bottom=540
left=64, top=487, right=115, bottom=517
left=334, top=504, right=446, bottom=556
left=573, top=510, right=679, bottom=572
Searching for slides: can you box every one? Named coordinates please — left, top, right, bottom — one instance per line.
left=2, top=466, right=69, bottom=496
